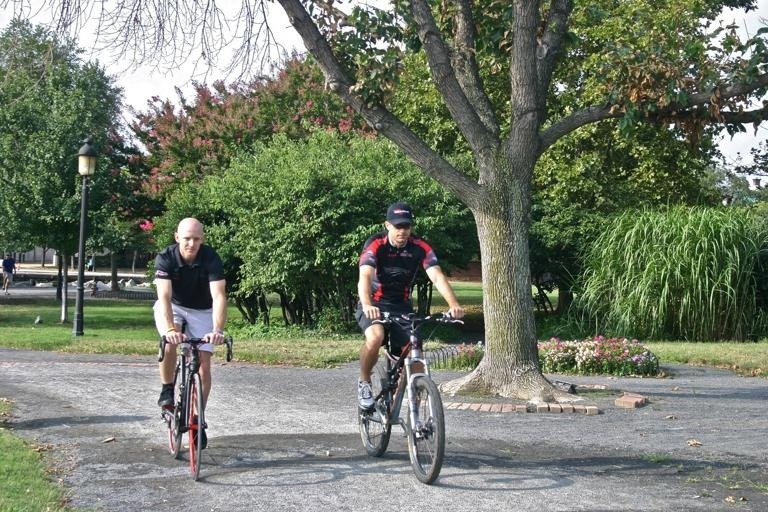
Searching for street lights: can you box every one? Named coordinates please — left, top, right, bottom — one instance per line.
left=72, top=136, right=99, bottom=338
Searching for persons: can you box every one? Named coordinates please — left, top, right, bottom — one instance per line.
left=1, top=253, right=16, bottom=296
left=354, top=202, right=466, bottom=443
left=151, top=216, right=228, bottom=451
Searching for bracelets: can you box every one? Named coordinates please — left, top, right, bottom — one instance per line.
left=166, top=328, right=175, bottom=333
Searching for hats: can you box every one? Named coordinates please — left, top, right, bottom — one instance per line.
left=386, top=203, right=413, bottom=225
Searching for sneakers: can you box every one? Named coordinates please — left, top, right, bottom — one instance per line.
left=358, top=378, right=375, bottom=411
left=158, top=386, right=173, bottom=406
left=193, top=420, right=207, bottom=449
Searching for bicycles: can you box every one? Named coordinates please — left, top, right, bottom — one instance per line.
left=156, top=333, right=234, bottom=482
left=357, top=309, right=465, bottom=485
